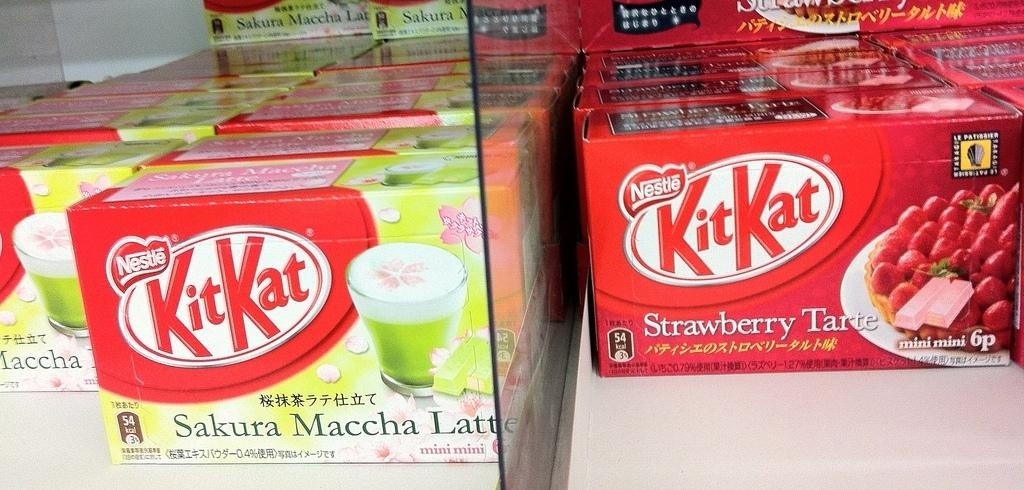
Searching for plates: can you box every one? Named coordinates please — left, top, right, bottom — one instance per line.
left=756, top=41, right=939, bottom=112
left=836, top=226, right=1011, bottom=366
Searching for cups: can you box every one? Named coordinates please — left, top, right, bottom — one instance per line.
left=379, top=73, right=475, bottom=183
left=344, top=240, right=468, bottom=386
left=8, top=212, right=95, bottom=341
